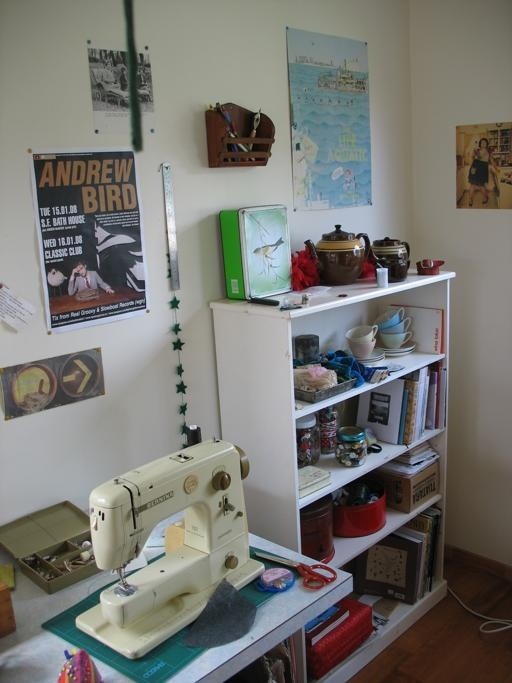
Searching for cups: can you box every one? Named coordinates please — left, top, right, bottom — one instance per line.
left=321, top=419, right=336, bottom=453
left=345, top=325, right=378, bottom=356
left=375, top=268, right=389, bottom=288
left=374, top=307, right=413, bottom=348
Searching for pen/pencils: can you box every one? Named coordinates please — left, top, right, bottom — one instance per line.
left=210, top=103, right=257, bottom=162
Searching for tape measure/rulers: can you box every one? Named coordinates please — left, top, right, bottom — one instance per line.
left=161, top=163, right=181, bottom=290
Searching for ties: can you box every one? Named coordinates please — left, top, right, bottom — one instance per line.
left=85, top=277, right=90, bottom=288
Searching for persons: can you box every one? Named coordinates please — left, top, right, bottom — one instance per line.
left=119, top=67, right=150, bottom=96
left=143, top=46, right=150, bottom=68
left=68, top=258, right=115, bottom=297
left=468, top=137, right=500, bottom=206
left=97, top=59, right=115, bottom=90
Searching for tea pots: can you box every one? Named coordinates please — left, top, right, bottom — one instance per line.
left=367, top=236, right=410, bottom=260
left=302, top=225, right=371, bottom=286
left=373, top=257, right=410, bottom=280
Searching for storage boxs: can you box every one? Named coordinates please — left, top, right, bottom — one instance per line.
left=370, top=461, right=440, bottom=513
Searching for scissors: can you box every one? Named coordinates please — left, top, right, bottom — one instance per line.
left=255, top=551, right=338, bottom=590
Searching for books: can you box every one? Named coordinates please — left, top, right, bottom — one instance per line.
left=355, top=360, right=446, bottom=445
left=390, top=304, right=443, bottom=354
left=353, top=507, right=442, bottom=604
left=305, top=602, right=349, bottom=646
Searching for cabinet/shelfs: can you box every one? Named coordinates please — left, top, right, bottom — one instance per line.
left=208, top=268, right=457, bottom=683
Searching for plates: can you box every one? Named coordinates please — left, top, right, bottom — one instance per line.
left=377, top=342, right=416, bottom=356
left=343, top=349, right=385, bottom=364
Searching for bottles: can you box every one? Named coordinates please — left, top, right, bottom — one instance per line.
left=297, top=414, right=319, bottom=468
left=335, top=427, right=368, bottom=466
